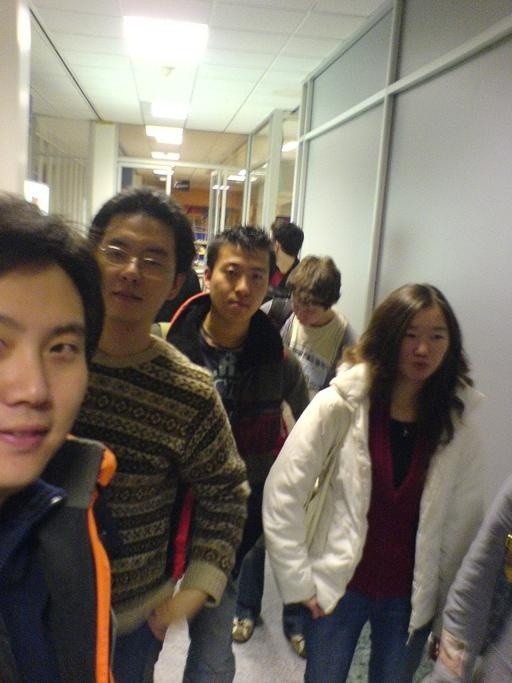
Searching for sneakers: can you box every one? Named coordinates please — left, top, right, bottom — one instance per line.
left=231, top=616, right=254, bottom=644
left=288, top=629, right=312, bottom=662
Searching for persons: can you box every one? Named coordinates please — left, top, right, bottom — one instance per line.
left=147, top=224, right=310, bottom=683
left=260, top=221, right=305, bottom=301
left=421, top=473, right=512, bottom=683
left=66, top=182, right=251, bottom=683
left=262, top=283, right=489, bottom=683
left=0, top=194, right=119, bottom=683
left=232, top=254, right=358, bottom=658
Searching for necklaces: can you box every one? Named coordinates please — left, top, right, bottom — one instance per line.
left=199, top=320, right=247, bottom=350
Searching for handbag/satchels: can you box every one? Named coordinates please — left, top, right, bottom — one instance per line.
left=296, top=410, right=353, bottom=556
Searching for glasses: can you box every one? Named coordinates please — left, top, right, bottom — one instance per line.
left=90, top=242, right=174, bottom=275
